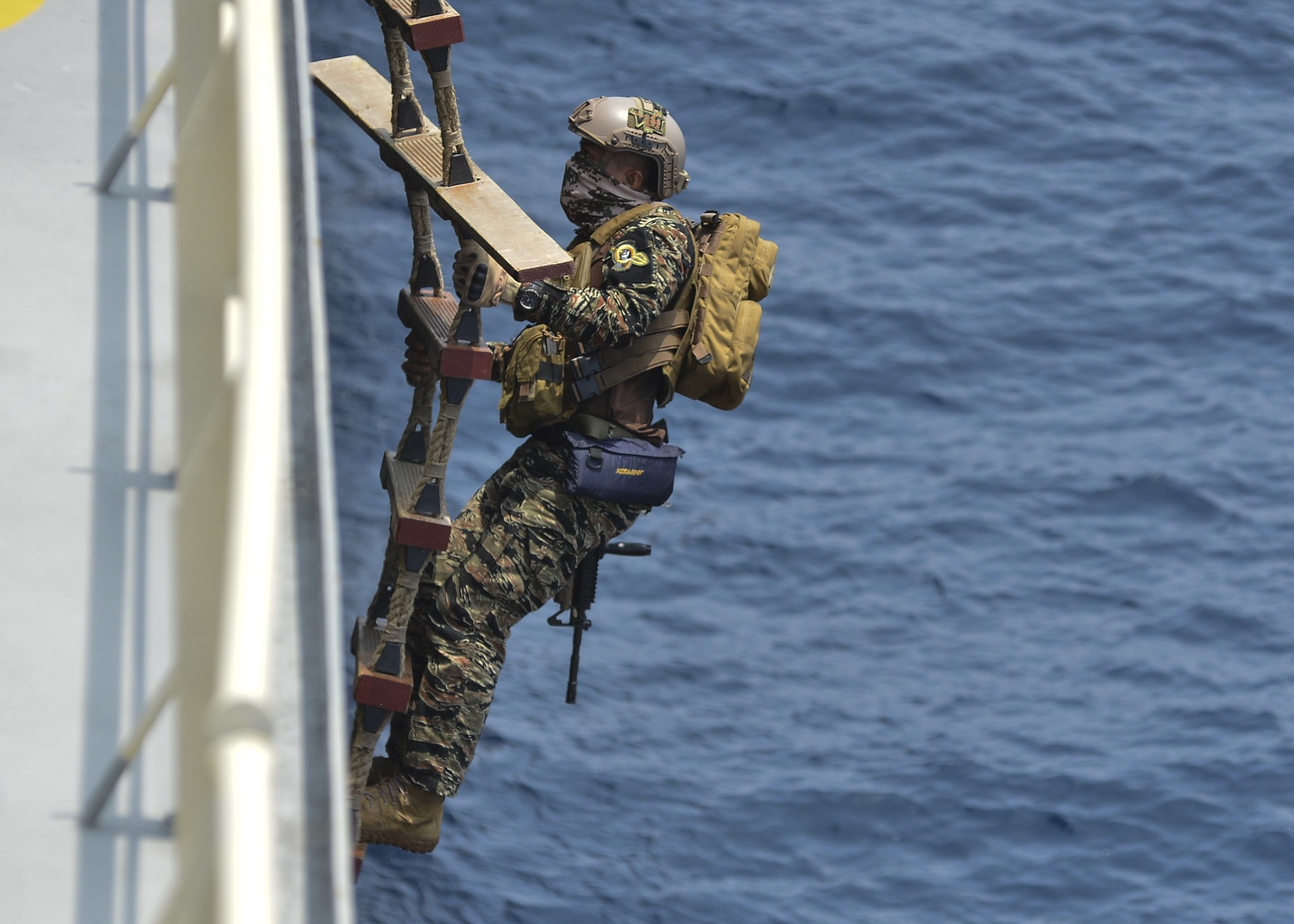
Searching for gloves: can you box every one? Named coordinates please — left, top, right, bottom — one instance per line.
left=452, top=237, right=522, bottom=308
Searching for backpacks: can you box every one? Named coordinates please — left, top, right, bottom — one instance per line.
left=591, top=200, right=778, bottom=410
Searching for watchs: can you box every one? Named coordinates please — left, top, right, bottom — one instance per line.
left=513, top=281, right=543, bottom=322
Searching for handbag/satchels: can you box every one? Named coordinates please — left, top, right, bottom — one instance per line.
left=563, top=437, right=684, bottom=507
left=498, top=324, right=577, bottom=437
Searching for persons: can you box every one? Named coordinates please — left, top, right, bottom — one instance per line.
left=347, top=98, right=700, bottom=855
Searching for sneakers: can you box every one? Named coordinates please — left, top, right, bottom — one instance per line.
left=357, top=774, right=445, bottom=854
left=366, top=755, right=402, bottom=786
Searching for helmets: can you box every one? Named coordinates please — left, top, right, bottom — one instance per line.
left=567, top=97, right=689, bottom=198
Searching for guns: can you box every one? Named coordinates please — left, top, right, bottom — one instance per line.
left=546, top=539, right=666, bottom=707
